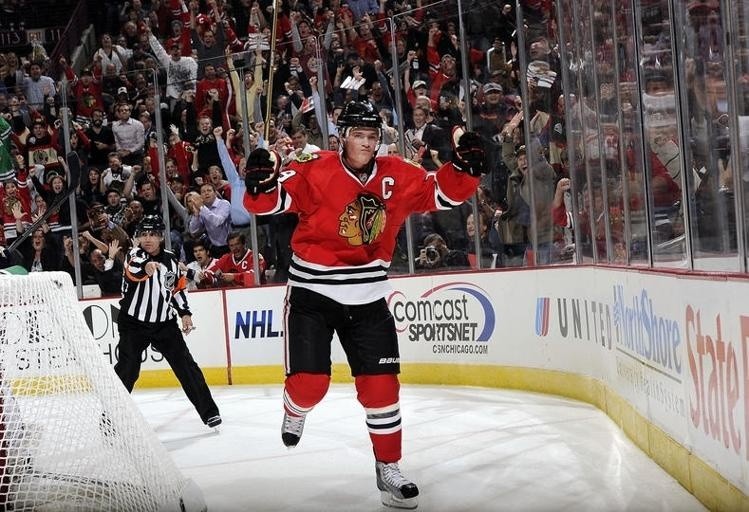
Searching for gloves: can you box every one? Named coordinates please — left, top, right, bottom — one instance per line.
left=244, top=145, right=280, bottom=194
left=451, top=130, right=501, bottom=176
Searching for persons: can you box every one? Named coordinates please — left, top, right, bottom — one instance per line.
left=100, top=214, right=222, bottom=436
left=243, top=101, right=484, bottom=500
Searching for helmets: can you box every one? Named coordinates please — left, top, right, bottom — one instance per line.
left=336, top=100, right=384, bottom=129
left=136, top=213, right=168, bottom=232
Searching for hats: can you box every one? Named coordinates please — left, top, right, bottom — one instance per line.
left=482, top=81, right=504, bottom=94
left=117, top=86, right=128, bottom=97
left=411, top=79, right=427, bottom=90
left=81, top=68, right=92, bottom=77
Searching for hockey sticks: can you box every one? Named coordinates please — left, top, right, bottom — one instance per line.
left=7, top=153, right=80, bottom=254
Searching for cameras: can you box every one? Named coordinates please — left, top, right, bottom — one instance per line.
left=425, top=246, right=437, bottom=261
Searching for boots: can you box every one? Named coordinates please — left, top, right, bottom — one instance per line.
left=279, top=409, right=305, bottom=447
left=207, top=414, right=223, bottom=428
left=372, top=461, right=418, bottom=502
